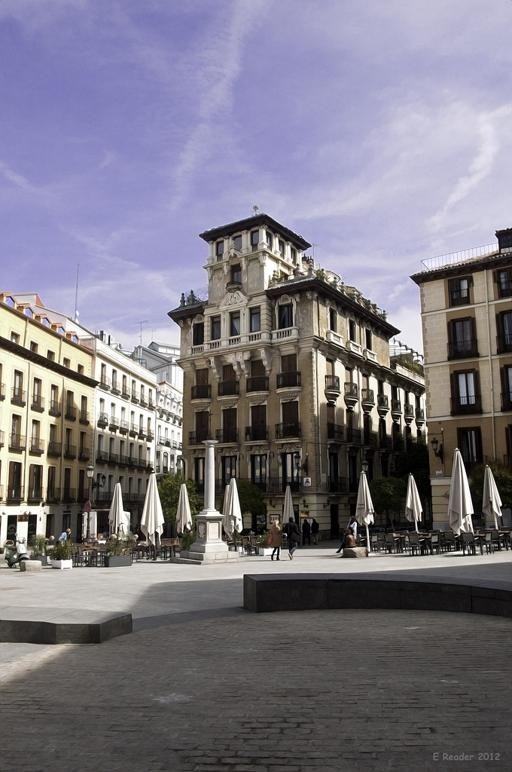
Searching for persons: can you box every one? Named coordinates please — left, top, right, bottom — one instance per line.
left=53, top=527, right=72, bottom=546
left=311, top=518, right=319, bottom=545
left=267, top=519, right=282, bottom=560
left=335, top=527, right=354, bottom=552
left=348, top=516, right=358, bottom=544
left=284, top=517, right=301, bottom=560
left=302, top=519, right=310, bottom=545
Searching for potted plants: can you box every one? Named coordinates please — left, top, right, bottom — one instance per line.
left=104, top=540, right=136, bottom=566
left=51, top=539, right=74, bottom=569
left=258, top=530, right=281, bottom=557
left=28, top=533, right=51, bottom=565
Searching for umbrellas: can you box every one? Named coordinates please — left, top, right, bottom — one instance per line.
left=140, top=470, right=166, bottom=552
left=481, top=464, right=502, bottom=529
left=280, top=482, right=296, bottom=534
left=447, top=447, right=475, bottom=540
left=174, top=483, right=192, bottom=536
left=354, top=470, right=376, bottom=549
left=227, top=477, right=243, bottom=533
left=107, top=482, right=126, bottom=538
left=404, top=472, right=424, bottom=531
left=222, top=484, right=235, bottom=540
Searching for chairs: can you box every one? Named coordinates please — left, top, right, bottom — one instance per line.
left=370, top=528, right=511, bottom=556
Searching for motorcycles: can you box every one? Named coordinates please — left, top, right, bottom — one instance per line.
left=3, top=539, right=29, bottom=567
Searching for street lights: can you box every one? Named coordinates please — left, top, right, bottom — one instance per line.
left=86, top=464, right=95, bottom=537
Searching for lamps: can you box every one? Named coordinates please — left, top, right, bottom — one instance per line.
left=430, top=436, right=444, bottom=463
left=294, top=452, right=309, bottom=476
